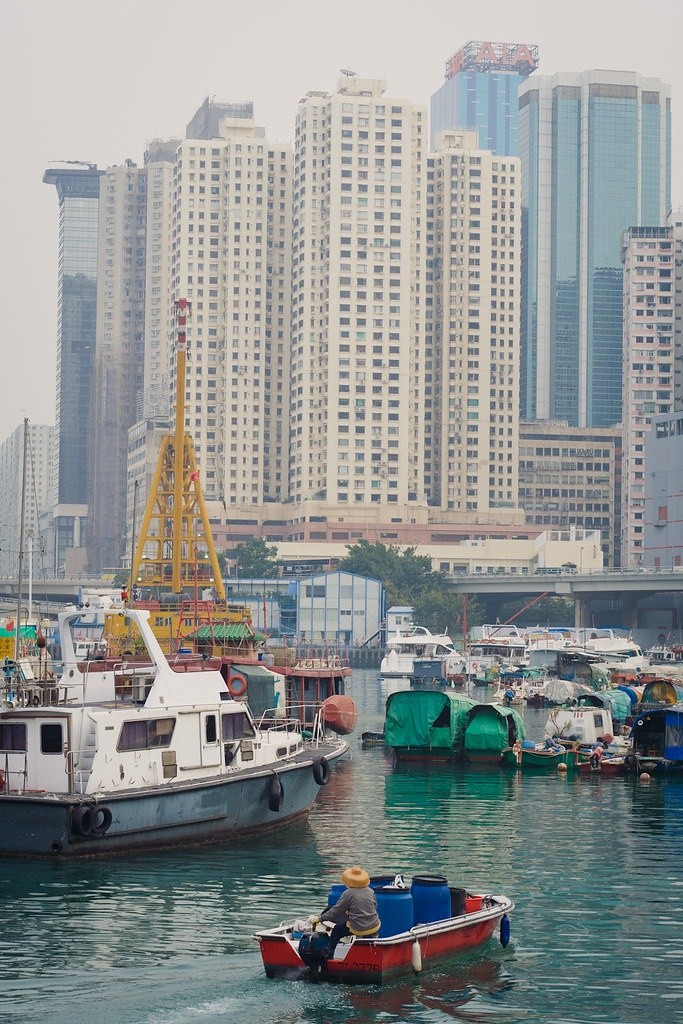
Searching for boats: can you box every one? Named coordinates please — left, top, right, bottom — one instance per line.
left=359, top=617, right=683, bottom=777
left=0, top=419, right=358, bottom=862
left=255, top=892, right=516, bottom=985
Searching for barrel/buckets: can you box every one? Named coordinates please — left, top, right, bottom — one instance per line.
left=372, top=886, right=414, bottom=938
left=368, top=875, right=404, bottom=888
left=411, top=875, right=451, bottom=927
left=262, top=652, right=274, bottom=667
left=328, top=885, right=348, bottom=906
left=449, top=887, right=466, bottom=917
left=466, top=896, right=483, bottom=913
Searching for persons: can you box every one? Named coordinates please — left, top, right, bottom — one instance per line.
left=623, top=748, right=642, bottom=778
left=589, top=745, right=604, bottom=770
left=309, top=866, right=382, bottom=961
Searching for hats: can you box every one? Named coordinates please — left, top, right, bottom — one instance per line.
left=342, top=866, right=370, bottom=887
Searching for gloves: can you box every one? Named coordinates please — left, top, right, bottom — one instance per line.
left=306, top=915, right=319, bottom=924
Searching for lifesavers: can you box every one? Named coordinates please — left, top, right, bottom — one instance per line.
left=269, top=778, right=284, bottom=812
left=313, top=756, right=330, bottom=785
left=72, top=806, right=112, bottom=836
left=472, top=663, right=477, bottom=668
left=227, top=674, right=247, bottom=696
